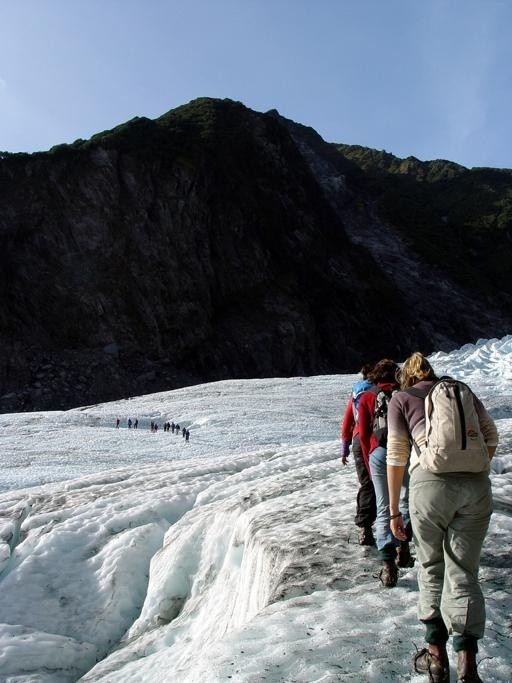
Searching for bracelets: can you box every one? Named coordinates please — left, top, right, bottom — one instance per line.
left=389, top=512, right=402, bottom=520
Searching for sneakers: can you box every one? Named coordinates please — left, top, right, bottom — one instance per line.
left=359, top=533, right=374, bottom=546
left=397, top=557, right=414, bottom=568
left=382, top=569, right=397, bottom=587
left=456, top=665, right=483, bottom=683
left=412, top=648, right=450, bottom=683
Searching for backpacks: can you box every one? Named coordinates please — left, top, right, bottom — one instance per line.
left=367, top=383, right=400, bottom=449
left=391, top=376, right=489, bottom=474
left=352, top=391, right=367, bottom=423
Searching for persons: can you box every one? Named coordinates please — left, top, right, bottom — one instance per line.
left=114, top=418, right=189, bottom=440
left=387, top=351, right=499, bottom=682
left=342, top=366, right=376, bottom=545
left=357, top=360, right=415, bottom=590
